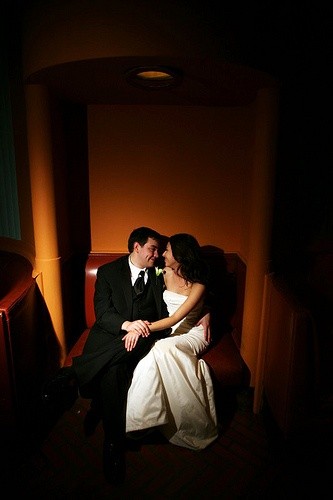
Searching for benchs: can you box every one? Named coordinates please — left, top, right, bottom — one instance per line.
left=60, top=253, right=251, bottom=385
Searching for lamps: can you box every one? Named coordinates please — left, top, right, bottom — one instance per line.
left=124, top=57, right=184, bottom=91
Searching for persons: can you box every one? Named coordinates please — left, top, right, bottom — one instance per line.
left=122, top=233, right=219, bottom=453
left=78, top=227, right=211, bottom=487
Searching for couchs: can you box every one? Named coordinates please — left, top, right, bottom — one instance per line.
left=0, top=250, right=36, bottom=423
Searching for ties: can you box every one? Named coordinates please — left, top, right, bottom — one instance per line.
left=134, top=271, right=145, bottom=295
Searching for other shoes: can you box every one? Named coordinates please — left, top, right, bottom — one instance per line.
left=83, top=399, right=102, bottom=430
left=102, top=436, right=129, bottom=487
